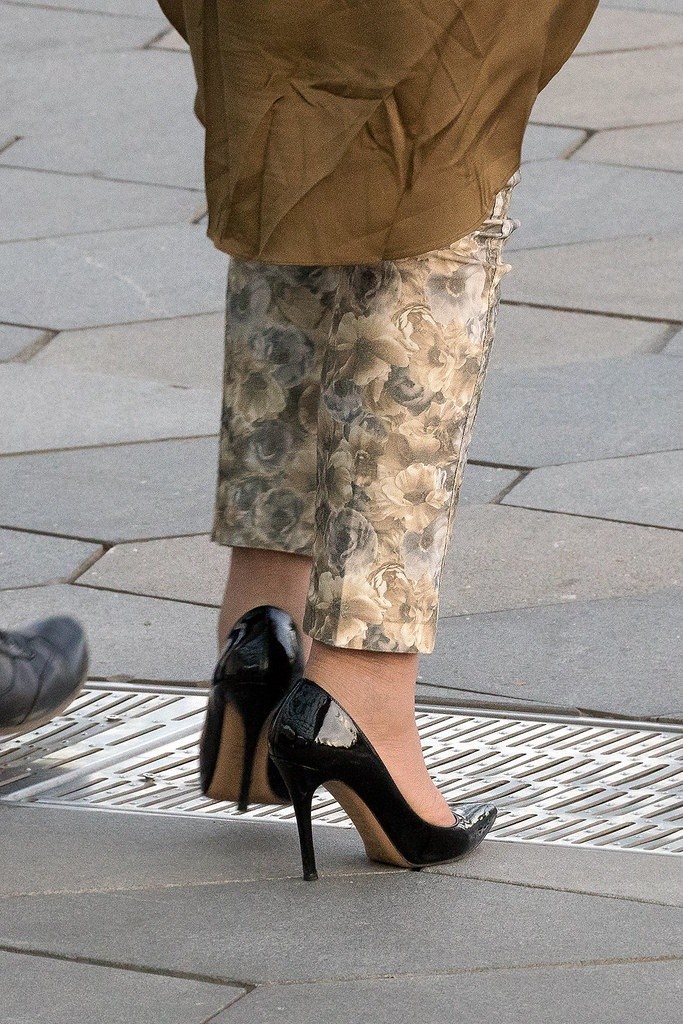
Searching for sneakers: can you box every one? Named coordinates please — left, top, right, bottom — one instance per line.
left=0, top=616, right=90, bottom=736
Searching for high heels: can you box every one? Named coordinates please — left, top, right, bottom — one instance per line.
left=199, top=604, right=304, bottom=812
left=266, top=680, right=498, bottom=881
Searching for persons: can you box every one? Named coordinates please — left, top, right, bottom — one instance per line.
left=155, top=0, right=599, bottom=880
left=0, top=613, right=92, bottom=735
